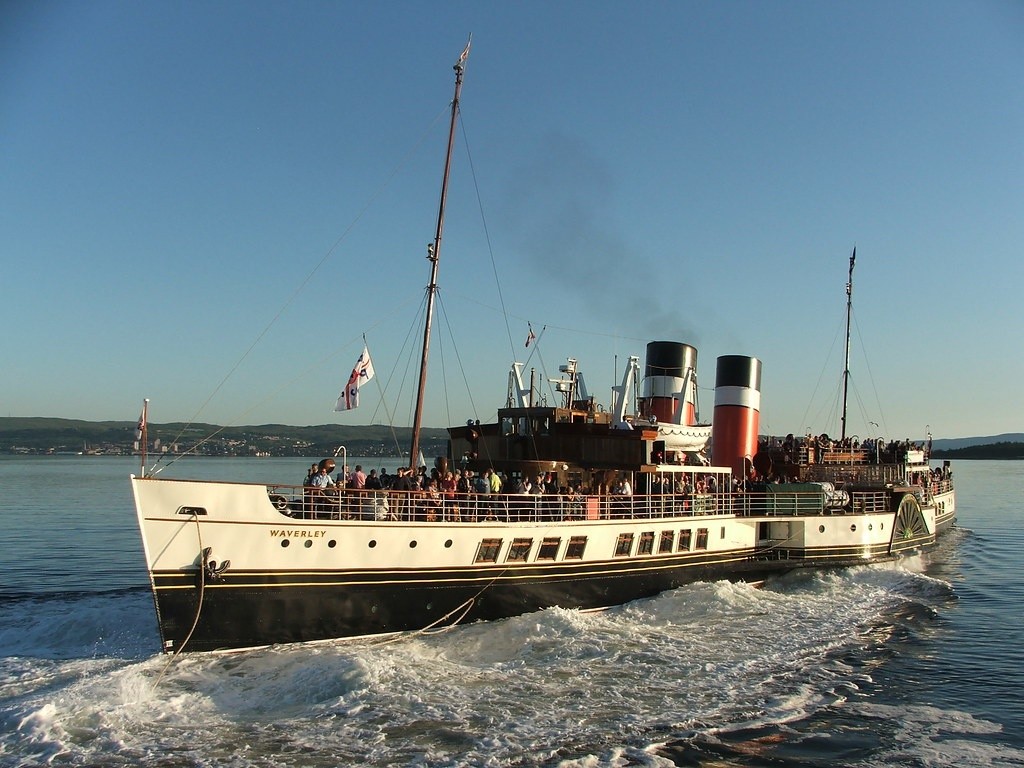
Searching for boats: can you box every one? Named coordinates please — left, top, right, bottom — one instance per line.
left=129, top=26, right=957, bottom=657
left=629, top=368, right=714, bottom=453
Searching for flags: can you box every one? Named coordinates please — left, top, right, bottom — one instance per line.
left=525, top=328, right=536, bottom=348
left=134, top=408, right=144, bottom=450
left=334, top=343, right=374, bottom=412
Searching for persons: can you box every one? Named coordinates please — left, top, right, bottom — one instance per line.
left=304, top=464, right=504, bottom=522
left=516, top=474, right=719, bottom=521
left=911, top=466, right=953, bottom=496
left=759, top=434, right=929, bottom=467
left=721, top=473, right=818, bottom=516
